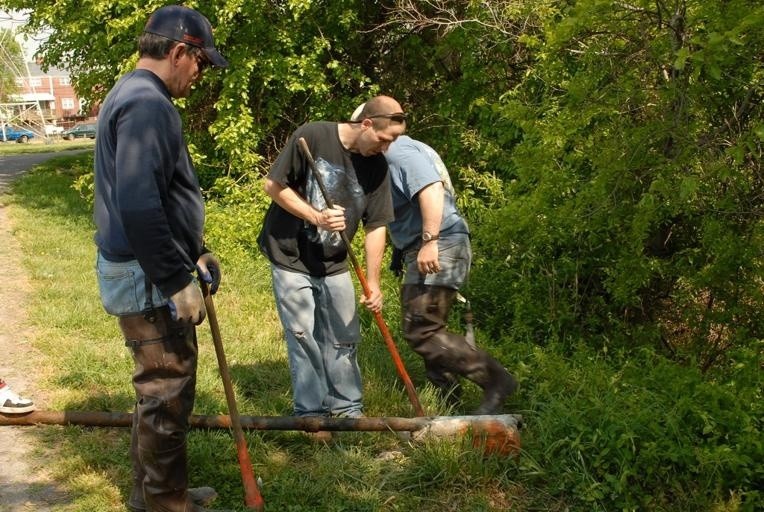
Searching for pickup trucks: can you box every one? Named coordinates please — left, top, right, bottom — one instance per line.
left=0, top=127, right=33, bottom=144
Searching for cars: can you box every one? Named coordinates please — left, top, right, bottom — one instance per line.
left=41, top=124, right=64, bottom=137
left=63, top=123, right=96, bottom=140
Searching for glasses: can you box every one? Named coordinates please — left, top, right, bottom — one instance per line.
left=192, top=52, right=211, bottom=73
left=369, top=113, right=409, bottom=122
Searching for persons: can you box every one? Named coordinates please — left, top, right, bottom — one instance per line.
left=256, top=96, right=408, bottom=442
left=94, top=6, right=228, bottom=511
left=349, top=103, right=517, bottom=415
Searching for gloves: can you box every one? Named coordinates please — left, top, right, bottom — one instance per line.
left=169, top=283, right=207, bottom=327
left=195, top=254, right=221, bottom=294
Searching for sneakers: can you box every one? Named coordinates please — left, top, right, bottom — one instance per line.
left=0, top=379, right=35, bottom=414
left=129, top=486, right=218, bottom=512
left=477, top=369, right=518, bottom=415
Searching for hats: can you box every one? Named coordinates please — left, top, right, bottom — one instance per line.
left=143, top=6, right=228, bottom=67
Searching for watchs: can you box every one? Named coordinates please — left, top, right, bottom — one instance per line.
left=421, top=232, right=440, bottom=242
